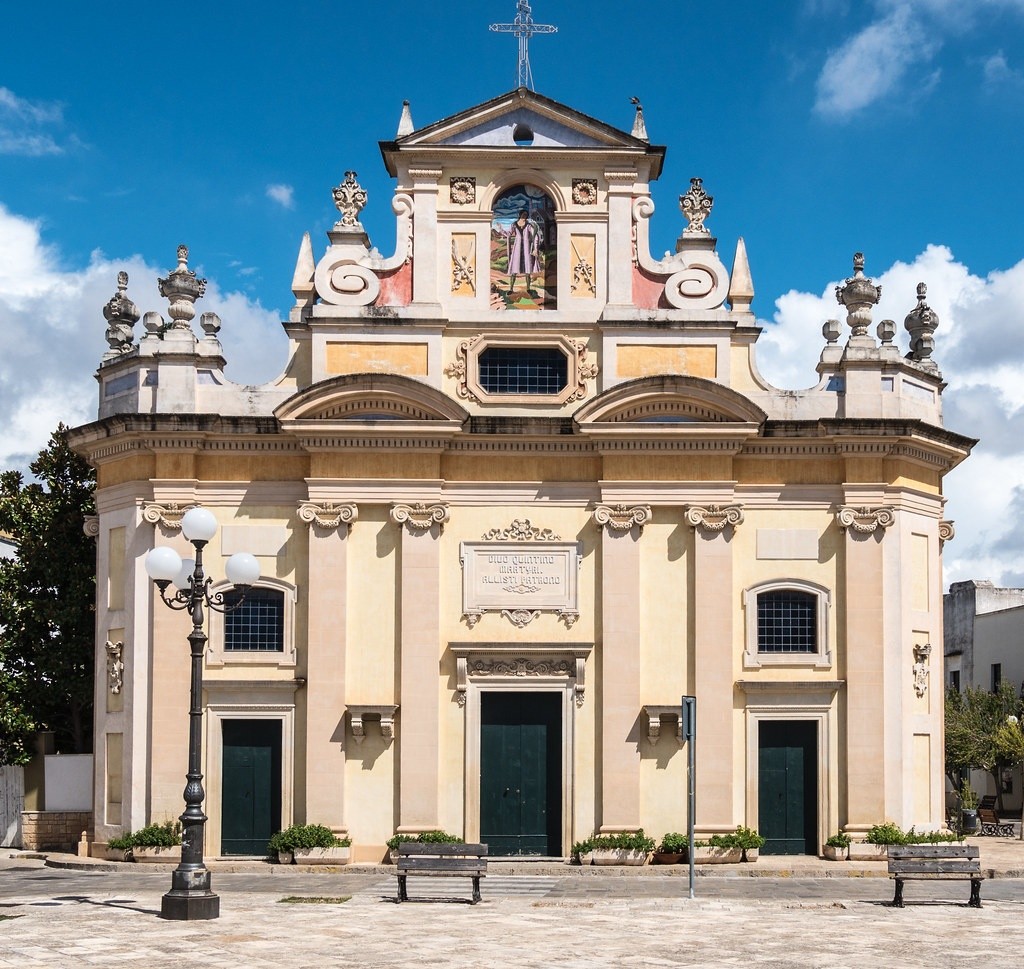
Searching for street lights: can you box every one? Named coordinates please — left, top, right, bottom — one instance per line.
left=144, top=507, right=261, bottom=920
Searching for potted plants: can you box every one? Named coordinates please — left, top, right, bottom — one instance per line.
left=104, top=837, right=132, bottom=861
left=847, top=821, right=967, bottom=861
left=288, top=823, right=350, bottom=864
left=687, top=825, right=745, bottom=863
left=592, top=830, right=656, bottom=866
left=821, top=830, right=852, bottom=861
left=385, top=834, right=465, bottom=864
left=960, top=778, right=976, bottom=831
left=570, top=837, right=592, bottom=865
left=131, top=820, right=182, bottom=863
left=266, top=828, right=294, bottom=864
left=653, top=832, right=687, bottom=864
left=739, top=826, right=766, bottom=861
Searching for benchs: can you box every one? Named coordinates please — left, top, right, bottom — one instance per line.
left=390, top=842, right=488, bottom=904
left=945, top=807, right=962, bottom=830
left=976, top=795, right=1015, bottom=837
left=886, top=845, right=985, bottom=909
